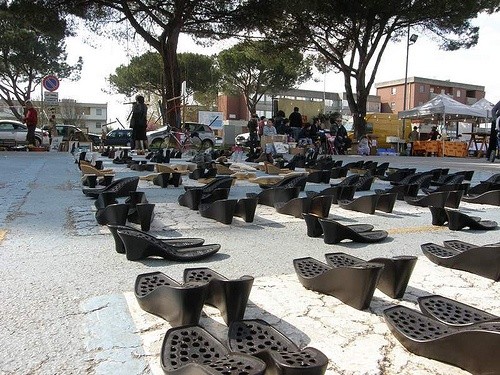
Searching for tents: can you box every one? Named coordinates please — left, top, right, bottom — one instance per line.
left=398, top=95, right=495, bottom=158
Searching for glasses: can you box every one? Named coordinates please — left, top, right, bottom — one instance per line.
left=335, top=120, right=342, bottom=122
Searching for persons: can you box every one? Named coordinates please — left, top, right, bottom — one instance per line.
left=18, top=100, right=38, bottom=149
left=247, top=106, right=370, bottom=157
left=407, top=126, right=442, bottom=157
left=129, top=95, right=148, bottom=150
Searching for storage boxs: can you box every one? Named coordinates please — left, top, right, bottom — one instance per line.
left=261, top=135, right=304, bottom=154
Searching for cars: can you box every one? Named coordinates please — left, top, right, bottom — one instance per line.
left=234, top=131, right=261, bottom=148
left=146, top=122, right=216, bottom=152
left=103, top=129, right=134, bottom=147
left=41, top=125, right=93, bottom=147
left=0, top=119, right=50, bottom=151
left=84, top=133, right=102, bottom=146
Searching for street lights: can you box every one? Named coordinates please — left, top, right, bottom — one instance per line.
left=402, top=25, right=419, bottom=140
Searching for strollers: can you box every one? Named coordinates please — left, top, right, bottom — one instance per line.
left=487, top=100, right=500, bottom=161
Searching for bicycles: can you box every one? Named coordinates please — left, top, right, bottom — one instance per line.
left=150, top=123, right=204, bottom=153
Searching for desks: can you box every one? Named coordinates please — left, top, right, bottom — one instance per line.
left=411, top=140, right=468, bottom=158
left=462, top=132, right=491, bottom=158
